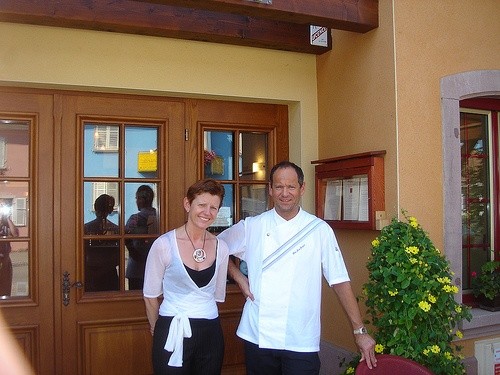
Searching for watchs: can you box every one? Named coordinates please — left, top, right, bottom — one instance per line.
left=353, top=327, right=368, bottom=335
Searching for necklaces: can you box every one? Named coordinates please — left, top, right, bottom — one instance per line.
left=185, top=223, right=207, bottom=263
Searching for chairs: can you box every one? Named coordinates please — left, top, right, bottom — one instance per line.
left=354, top=355, right=436, bottom=375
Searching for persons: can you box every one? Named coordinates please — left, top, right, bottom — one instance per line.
left=83, top=195, right=120, bottom=292
left=125, top=184, right=159, bottom=290
left=142, top=178, right=229, bottom=375
left=0, top=199, right=19, bottom=296
left=216, top=162, right=378, bottom=375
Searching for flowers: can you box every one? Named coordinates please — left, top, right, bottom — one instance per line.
left=338, top=207, right=475, bottom=375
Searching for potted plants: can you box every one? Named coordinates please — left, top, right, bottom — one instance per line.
left=471, top=261, right=500, bottom=312
